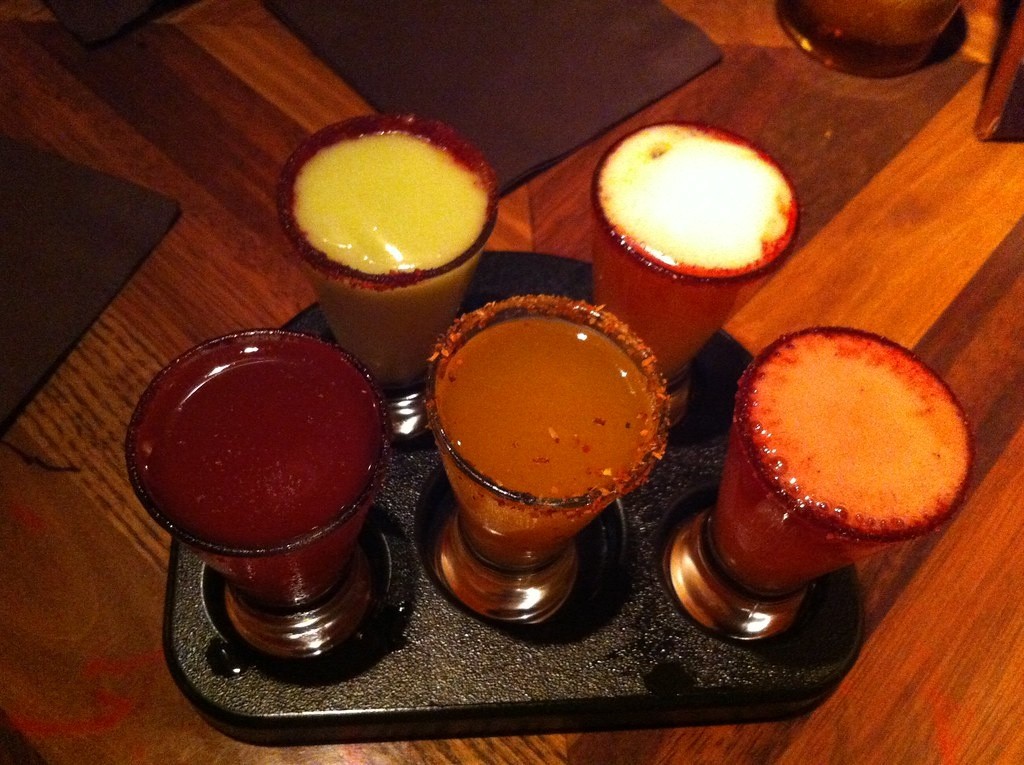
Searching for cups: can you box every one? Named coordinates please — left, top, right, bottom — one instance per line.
left=275, top=112, right=498, bottom=442
left=124, top=328, right=387, bottom=657
left=773, top=0, right=966, bottom=78
left=426, top=291, right=670, bottom=623
left=655, top=323, right=973, bottom=642
left=588, top=120, right=801, bottom=431
